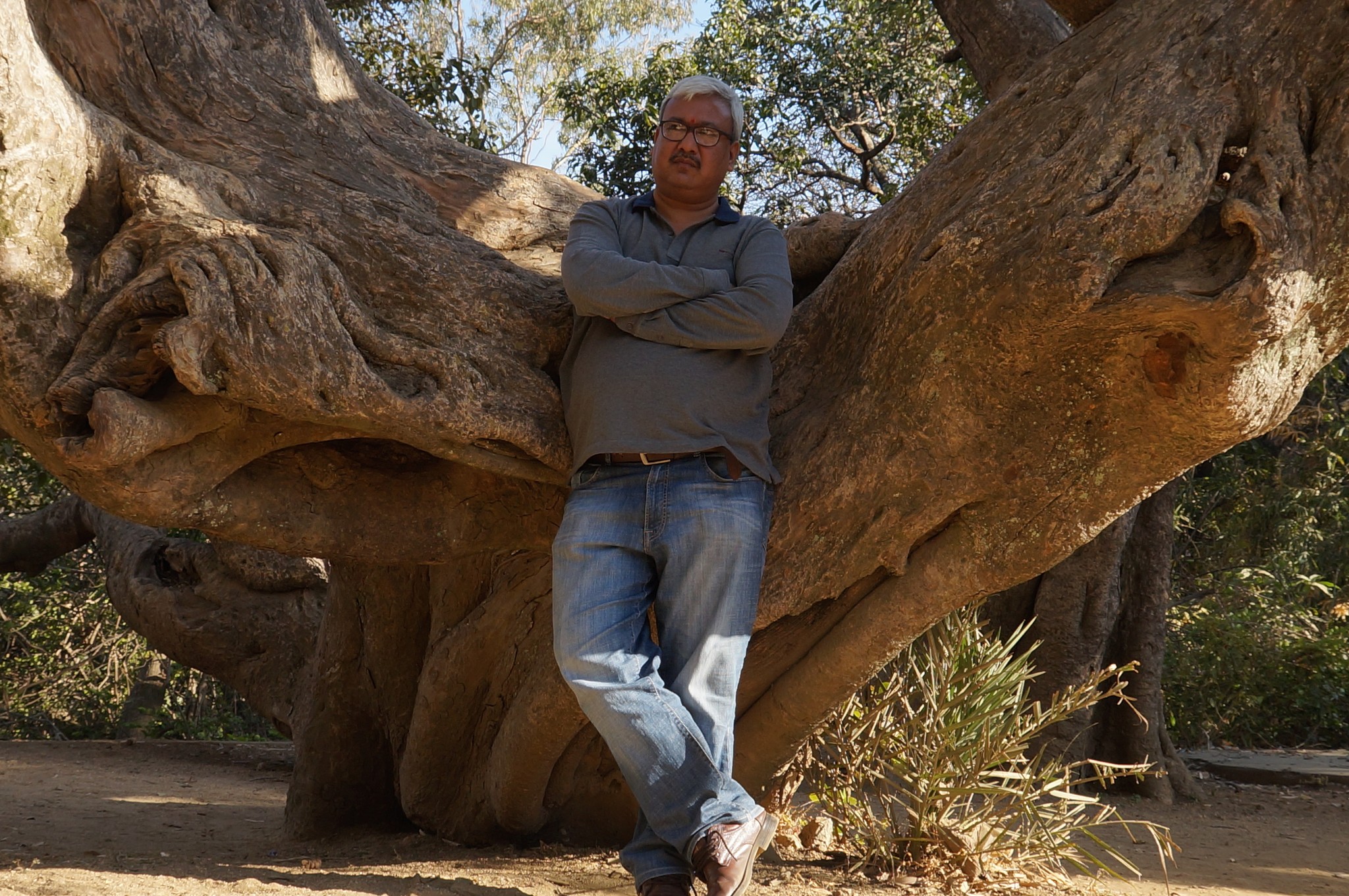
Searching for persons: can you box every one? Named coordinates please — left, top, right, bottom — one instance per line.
left=554, top=74, right=798, bottom=895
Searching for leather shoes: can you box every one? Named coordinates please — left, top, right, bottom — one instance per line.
left=692, top=804, right=780, bottom=896
left=586, top=445, right=742, bottom=480
left=640, top=874, right=692, bottom=896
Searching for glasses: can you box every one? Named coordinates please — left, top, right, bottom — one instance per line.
left=659, top=120, right=733, bottom=147
